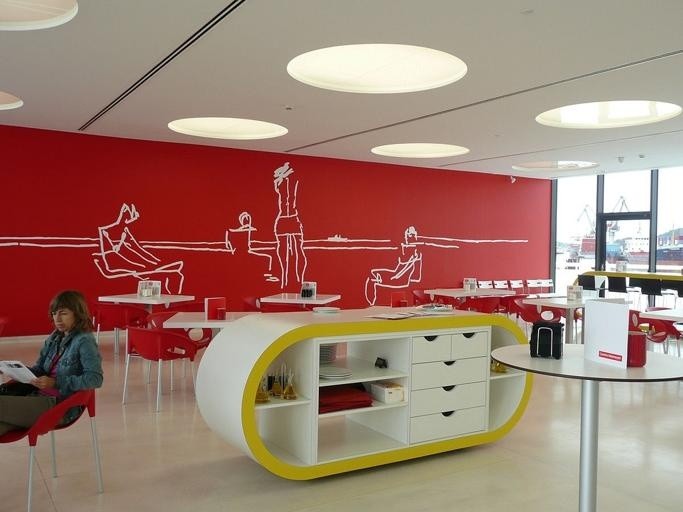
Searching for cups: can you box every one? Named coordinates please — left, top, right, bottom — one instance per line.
left=217, top=307, right=227, bottom=321
left=301, top=288, right=313, bottom=298
left=141, top=288, right=153, bottom=297
left=628, top=330, right=646, bottom=366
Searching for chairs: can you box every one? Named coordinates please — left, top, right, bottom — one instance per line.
left=94, top=297, right=310, bottom=412
left=1, top=379, right=103, bottom=512
left=390, top=278, right=683, bottom=358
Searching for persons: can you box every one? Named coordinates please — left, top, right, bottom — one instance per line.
left=0, top=290, right=103, bottom=441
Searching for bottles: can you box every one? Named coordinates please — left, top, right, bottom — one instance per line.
left=256, top=370, right=297, bottom=403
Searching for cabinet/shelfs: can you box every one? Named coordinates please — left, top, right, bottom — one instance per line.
left=195, top=308, right=534, bottom=480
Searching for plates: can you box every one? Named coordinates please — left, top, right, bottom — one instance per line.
left=313, top=307, right=454, bottom=320
left=319, top=342, right=353, bottom=380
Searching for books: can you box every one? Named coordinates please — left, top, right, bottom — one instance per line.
left=0, top=360, right=38, bottom=385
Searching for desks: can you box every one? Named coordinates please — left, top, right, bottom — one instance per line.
left=490, top=342, right=682, bottom=510
left=259, top=293, right=342, bottom=309
left=98, top=293, right=195, bottom=315
